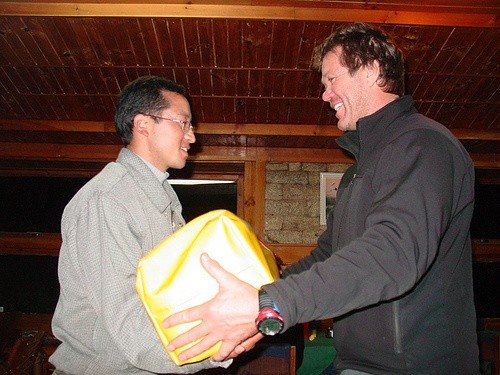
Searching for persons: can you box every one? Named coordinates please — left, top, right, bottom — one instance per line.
left=43, top=72, right=269, bottom=375
left=164, top=23, right=481, bottom=375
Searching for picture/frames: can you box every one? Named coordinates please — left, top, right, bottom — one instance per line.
left=320, top=172, right=344, bottom=226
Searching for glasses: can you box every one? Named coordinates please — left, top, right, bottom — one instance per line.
left=131, top=113, right=194, bottom=134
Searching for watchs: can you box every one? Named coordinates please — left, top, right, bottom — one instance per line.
left=255, top=288, right=285, bottom=338
